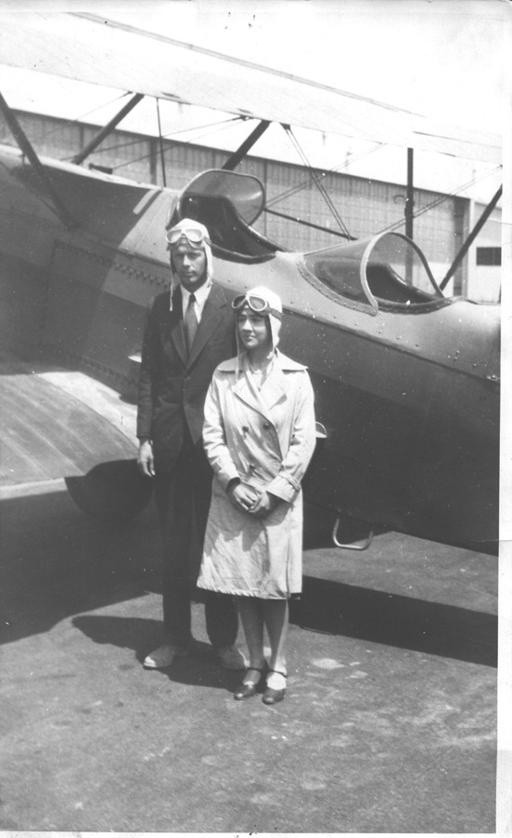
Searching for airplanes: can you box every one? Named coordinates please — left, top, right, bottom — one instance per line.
left=0, top=65, right=499, bottom=595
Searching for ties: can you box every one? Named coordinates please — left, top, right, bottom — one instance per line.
left=185, top=296, right=198, bottom=354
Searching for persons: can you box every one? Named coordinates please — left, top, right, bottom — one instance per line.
left=136, top=217, right=249, bottom=675
left=194, top=285, right=318, bottom=705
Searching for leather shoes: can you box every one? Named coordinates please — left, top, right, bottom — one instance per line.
left=216, top=645, right=251, bottom=671
left=143, top=645, right=189, bottom=670
left=263, top=662, right=287, bottom=704
left=233, top=658, right=269, bottom=700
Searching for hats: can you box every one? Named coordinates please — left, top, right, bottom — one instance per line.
left=236, top=286, right=283, bottom=347
left=168, top=219, right=212, bottom=287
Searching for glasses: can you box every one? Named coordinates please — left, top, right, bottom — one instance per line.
left=231, top=293, right=270, bottom=316
left=166, top=225, right=210, bottom=248
